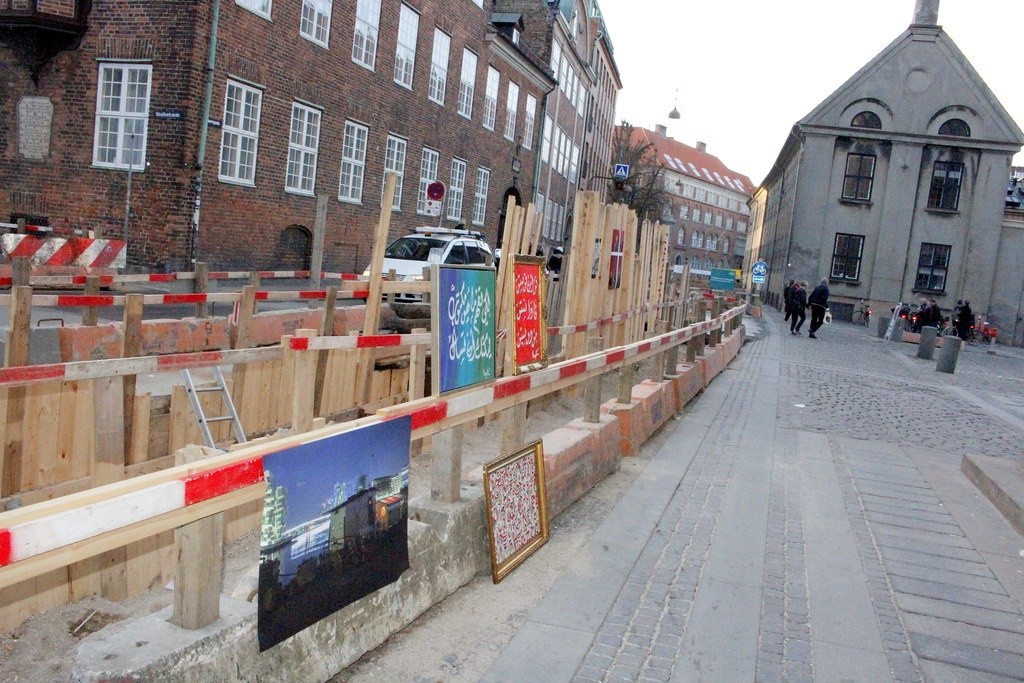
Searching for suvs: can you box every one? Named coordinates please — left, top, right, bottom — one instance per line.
left=361, top=227, right=498, bottom=304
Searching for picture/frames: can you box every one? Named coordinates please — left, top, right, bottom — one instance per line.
left=507, top=253, right=548, bottom=377
left=430, top=263, right=497, bottom=398
left=483, top=438, right=550, bottom=585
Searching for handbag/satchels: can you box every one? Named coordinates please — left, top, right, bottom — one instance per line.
left=968, top=314, right=975, bottom=325
left=823, top=312, right=832, bottom=324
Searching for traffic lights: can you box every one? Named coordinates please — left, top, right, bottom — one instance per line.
left=615, top=181, right=624, bottom=191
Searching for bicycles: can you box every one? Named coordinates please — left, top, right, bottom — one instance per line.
left=849, top=298, right=871, bottom=327
left=900, top=303, right=994, bottom=346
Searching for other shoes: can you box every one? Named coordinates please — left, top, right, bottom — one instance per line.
left=809, top=332, right=817, bottom=338
left=792, top=331, right=801, bottom=335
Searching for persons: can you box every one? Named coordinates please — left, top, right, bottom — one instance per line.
left=890, top=298, right=978, bottom=344
left=544, top=246, right=563, bottom=281
left=783, top=277, right=829, bottom=339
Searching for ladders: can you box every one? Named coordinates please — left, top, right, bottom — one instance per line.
left=180, top=366, right=247, bottom=449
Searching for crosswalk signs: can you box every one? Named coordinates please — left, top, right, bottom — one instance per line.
left=614, top=164, right=629, bottom=177
